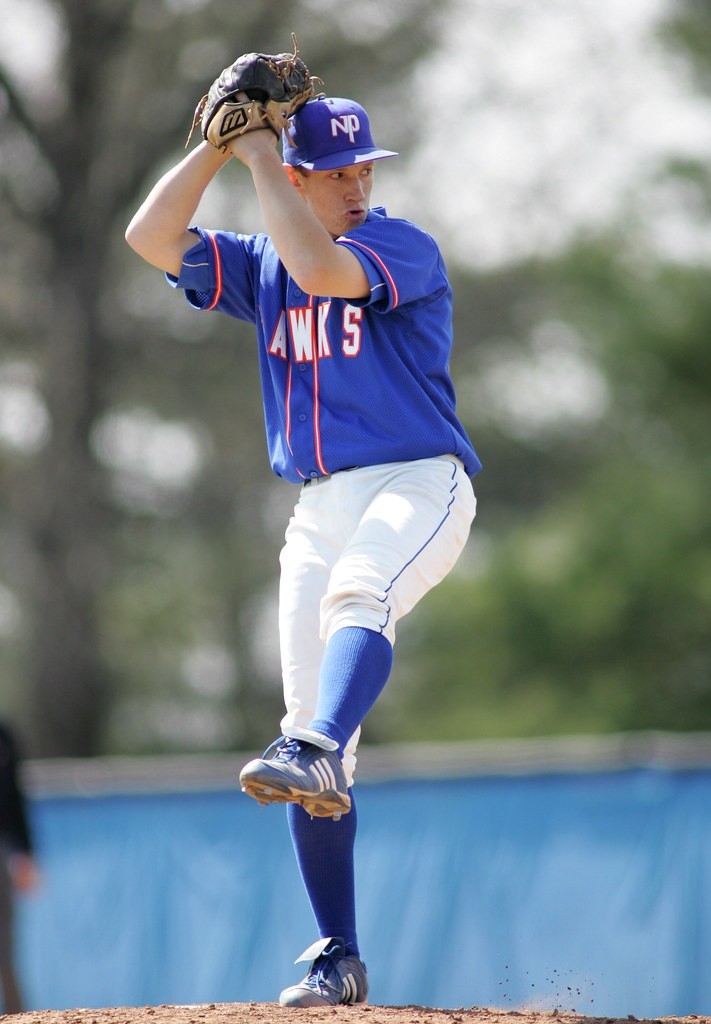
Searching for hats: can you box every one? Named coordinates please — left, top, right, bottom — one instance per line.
left=282, top=97, right=397, bottom=169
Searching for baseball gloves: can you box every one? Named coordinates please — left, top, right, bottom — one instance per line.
left=184, top=31, right=327, bottom=150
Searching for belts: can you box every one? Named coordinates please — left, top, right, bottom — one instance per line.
left=304, top=465, right=361, bottom=486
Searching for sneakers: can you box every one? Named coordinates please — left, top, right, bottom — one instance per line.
left=240, top=726, right=351, bottom=821
left=280, top=938, right=369, bottom=1008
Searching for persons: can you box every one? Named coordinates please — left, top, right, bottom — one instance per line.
left=125, top=33, right=481, bottom=1010
left=0, top=716, right=38, bottom=1015
